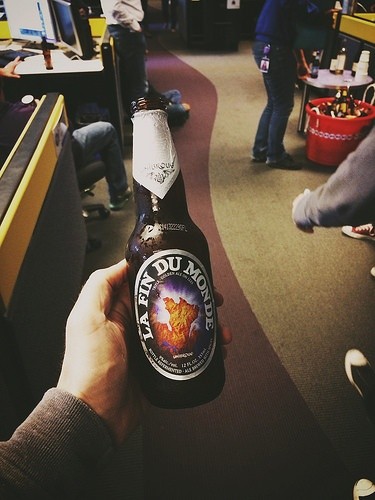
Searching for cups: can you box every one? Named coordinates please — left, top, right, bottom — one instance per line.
left=21, top=95, right=38, bottom=107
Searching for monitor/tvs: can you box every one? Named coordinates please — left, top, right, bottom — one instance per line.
left=50, top=0, right=86, bottom=57
left=3, top=0, right=60, bottom=44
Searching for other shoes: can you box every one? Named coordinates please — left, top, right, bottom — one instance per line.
left=253, top=152, right=303, bottom=170
left=353, top=478, right=375, bottom=500
left=107, top=187, right=136, bottom=216
left=344, top=349, right=375, bottom=417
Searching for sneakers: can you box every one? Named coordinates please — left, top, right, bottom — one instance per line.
left=342, top=223, right=374, bottom=241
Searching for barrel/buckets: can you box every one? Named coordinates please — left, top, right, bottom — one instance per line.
left=304, top=96, right=375, bottom=167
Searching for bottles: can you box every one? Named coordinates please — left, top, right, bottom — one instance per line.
left=308, top=49, right=320, bottom=78
left=41, top=36, right=53, bottom=69
left=124, top=96, right=227, bottom=411
left=309, top=89, right=372, bottom=118
left=335, top=48, right=346, bottom=74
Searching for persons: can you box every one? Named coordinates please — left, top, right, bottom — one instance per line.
left=291, top=119, right=375, bottom=500
left=98, top=0, right=193, bottom=133
left=0, top=55, right=134, bottom=213
left=247, top=0, right=342, bottom=171
left=0, top=237, right=235, bottom=500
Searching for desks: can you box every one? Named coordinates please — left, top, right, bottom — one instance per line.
left=297, top=70, right=372, bottom=132
left=0, top=38, right=107, bottom=80
